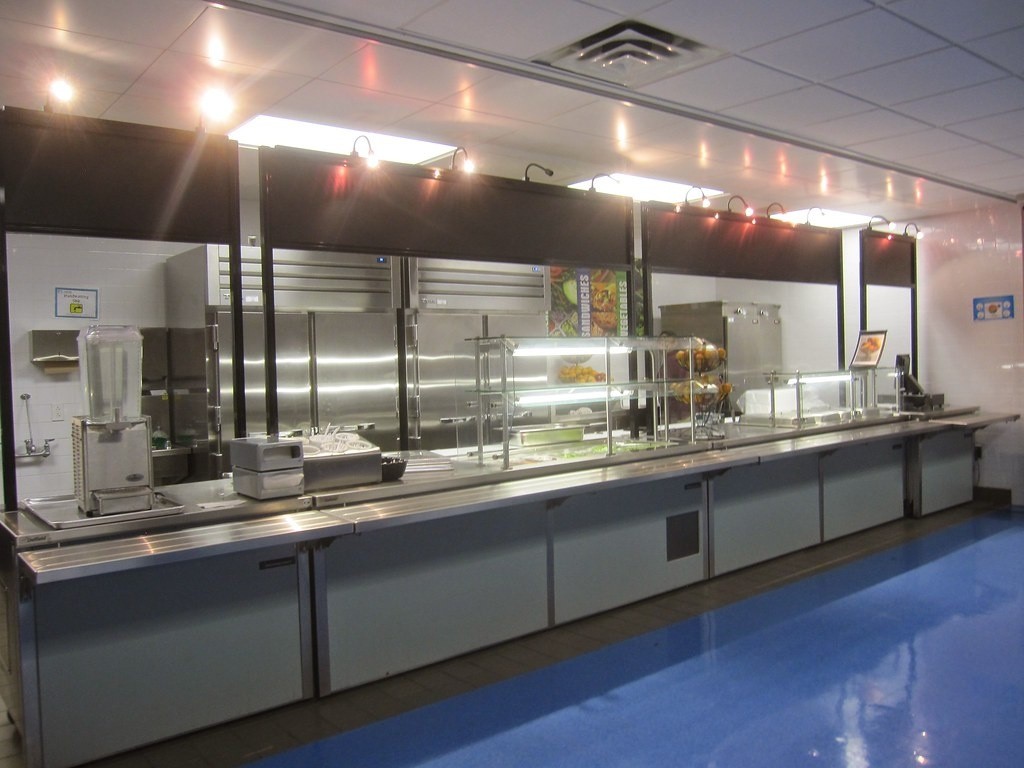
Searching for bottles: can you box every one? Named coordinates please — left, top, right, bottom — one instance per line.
left=166, top=441, right=172, bottom=450
left=184, top=423, right=197, bottom=445
left=192, top=439, right=197, bottom=447
left=152, top=425, right=168, bottom=449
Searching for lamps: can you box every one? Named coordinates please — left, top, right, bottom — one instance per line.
left=729, top=195, right=753, bottom=217
left=685, top=186, right=710, bottom=208
left=767, top=202, right=790, bottom=222
left=904, top=223, right=924, bottom=240
left=589, top=174, right=624, bottom=193
left=867, top=215, right=895, bottom=232
left=450, top=147, right=474, bottom=174
left=195, top=87, right=231, bottom=132
left=352, top=135, right=378, bottom=168
left=525, top=164, right=553, bottom=183
left=806, top=206, right=827, bottom=225
left=43, top=77, right=72, bottom=114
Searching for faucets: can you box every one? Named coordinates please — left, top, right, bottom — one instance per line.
left=24, top=438, right=37, bottom=456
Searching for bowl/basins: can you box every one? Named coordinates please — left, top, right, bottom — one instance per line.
left=380, top=456, right=409, bottom=480
left=287, top=432, right=371, bottom=455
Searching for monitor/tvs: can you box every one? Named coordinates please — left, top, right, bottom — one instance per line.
left=894, top=354, right=910, bottom=389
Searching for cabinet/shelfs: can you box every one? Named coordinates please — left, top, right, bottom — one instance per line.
left=466, top=335, right=730, bottom=468
left=731, top=367, right=905, bottom=427
